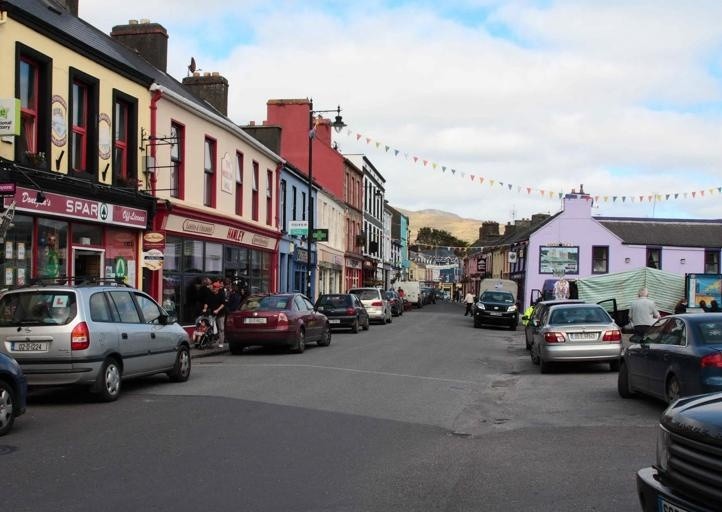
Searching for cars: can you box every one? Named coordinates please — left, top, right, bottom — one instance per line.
left=0, top=278, right=191, bottom=436
left=226, top=282, right=463, bottom=353
left=474, top=290, right=518, bottom=331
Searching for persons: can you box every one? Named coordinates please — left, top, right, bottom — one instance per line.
left=673, top=298, right=688, bottom=337
left=192, top=319, right=211, bottom=345
left=707, top=300, right=722, bottom=312
left=462, top=290, right=474, bottom=317
left=186, top=275, right=251, bottom=319
left=698, top=300, right=708, bottom=312
left=388, top=285, right=405, bottom=304
left=627, top=287, right=662, bottom=335
left=200, top=282, right=225, bottom=349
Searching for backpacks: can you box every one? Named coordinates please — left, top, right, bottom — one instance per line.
left=213, top=321, right=218, bottom=335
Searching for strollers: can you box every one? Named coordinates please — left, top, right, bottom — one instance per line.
left=193, top=310, right=219, bottom=350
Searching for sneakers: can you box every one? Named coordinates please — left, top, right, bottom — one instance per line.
left=464, top=312, right=474, bottom=317
left=212, top=335, right=229, bottom=348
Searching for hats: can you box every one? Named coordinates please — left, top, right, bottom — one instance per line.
left=212, top=282, right=220, bottom=288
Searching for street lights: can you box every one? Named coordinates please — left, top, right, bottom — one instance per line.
left=307, top=105, right=348, bottom=296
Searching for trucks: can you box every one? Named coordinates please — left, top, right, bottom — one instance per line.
left=530, top=267, right=686, bottom=334
